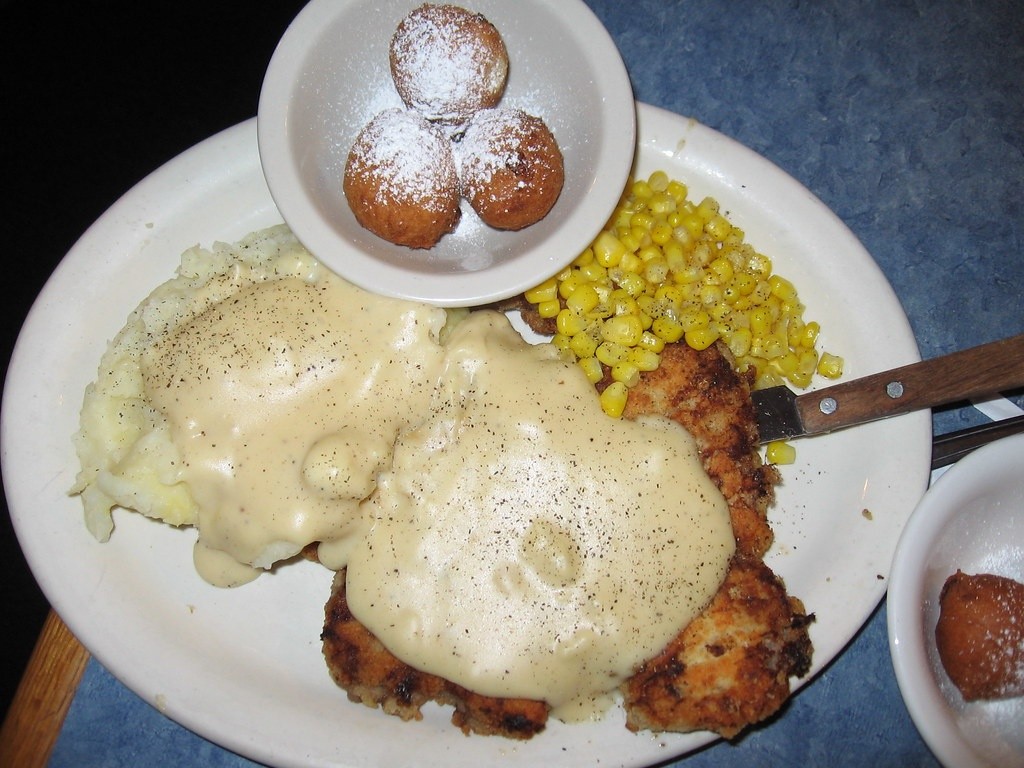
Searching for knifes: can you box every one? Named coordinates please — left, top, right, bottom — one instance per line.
left=751, top=332, right=1024, bottom=444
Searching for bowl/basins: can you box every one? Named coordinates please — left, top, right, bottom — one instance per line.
left=884, top=440, right=1021, bottom=768
left=253, top=2, right=635, bottom=308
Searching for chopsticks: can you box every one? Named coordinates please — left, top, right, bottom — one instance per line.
left=929, top=415, right=1024, bottom=470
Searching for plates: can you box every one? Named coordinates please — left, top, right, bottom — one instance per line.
left=4, top=95, right=942, bottom=765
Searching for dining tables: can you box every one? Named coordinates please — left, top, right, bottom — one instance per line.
left=1, top=1, right=1024, bottom=768
